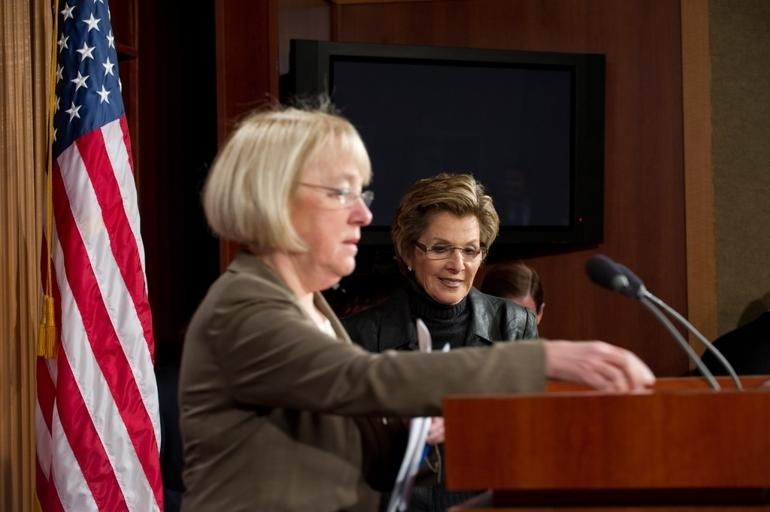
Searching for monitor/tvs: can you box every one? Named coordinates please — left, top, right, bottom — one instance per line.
left=289, top=38, right=606, bottom=265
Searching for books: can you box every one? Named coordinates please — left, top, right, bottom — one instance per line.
left=384, top=313, right=432, bottom=512
left=400, top=340, right=452, bottom=512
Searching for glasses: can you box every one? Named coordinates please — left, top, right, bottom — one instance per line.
left=295, top=180, right=374, bottom=211
left=413, top=240, right=486, bottom=260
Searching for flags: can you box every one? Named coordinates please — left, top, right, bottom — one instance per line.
left=31, top=0, right=173, bottom=511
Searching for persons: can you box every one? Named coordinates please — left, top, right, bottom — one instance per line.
left=477, top=260, right=547, bottom=332
left=177, top=94, right=656, bottom=512
left=335, top=170, right=539, bottom=512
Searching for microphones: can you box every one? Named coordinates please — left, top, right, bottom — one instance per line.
left=615, top=262, right=743, bottom=391
left=585, top=254, right=722, bottom=391
